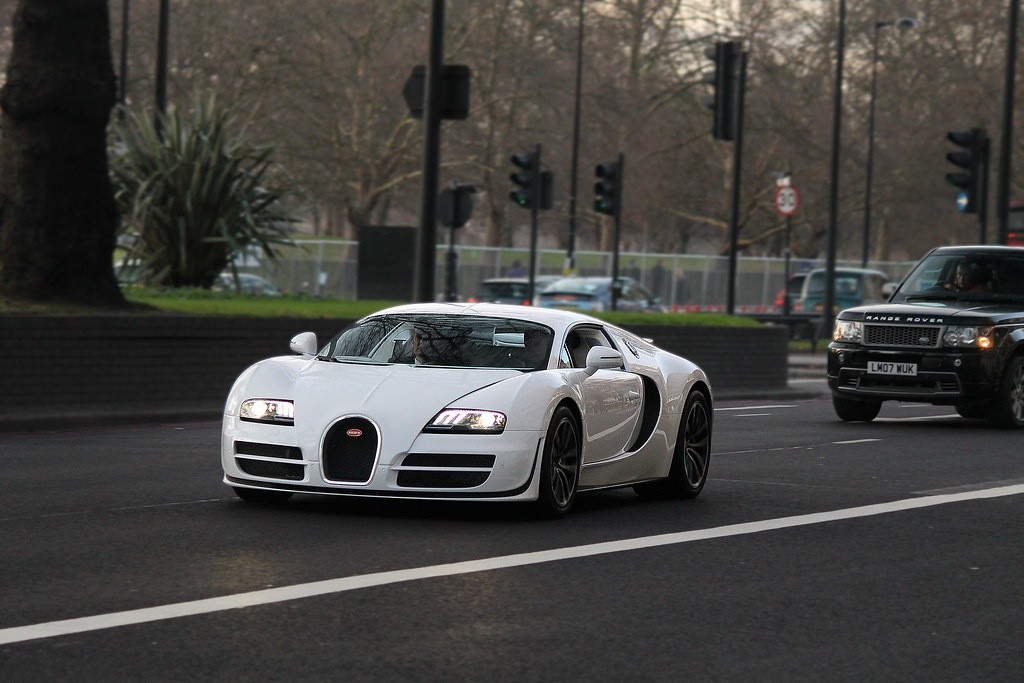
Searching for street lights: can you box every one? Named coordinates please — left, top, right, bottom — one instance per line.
left=860, top=18, right=917, bottom=268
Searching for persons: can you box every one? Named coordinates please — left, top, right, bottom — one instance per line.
left=509, top=329, right=550, bottom=368
left=946, top=261, right=993, bottom=293
left=390, top=321, right=444, bottom=365
left=506, top=259, right=527, bottom=277
left=622, top=259, right=691, bottom=309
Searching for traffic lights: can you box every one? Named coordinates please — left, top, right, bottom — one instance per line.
left=701, top=40, right=742, bottom=141
left=944, top=127, right=989, bottom=215
left=592, top=160, right=622, bottom=218
left=508, top=149, right=539, bottom=209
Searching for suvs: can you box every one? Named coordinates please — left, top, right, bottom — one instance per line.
left=828, top=245, right=1024, bottom=430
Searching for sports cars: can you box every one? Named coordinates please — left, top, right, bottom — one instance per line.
left=220, top=302, right=714, bottom=522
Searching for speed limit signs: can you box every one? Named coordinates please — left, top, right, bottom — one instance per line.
left=775, top=185, right=801, bottom=218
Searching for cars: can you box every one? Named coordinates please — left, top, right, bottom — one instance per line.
left=210, top=271, right=282, bottom=294
left=772, top=271, right=808, bottom=317
left=801, top=265, right=893, bottom=323
left=476, top=273, right=559, bottom=307
left=533, top=275, right=668, bottom=315
